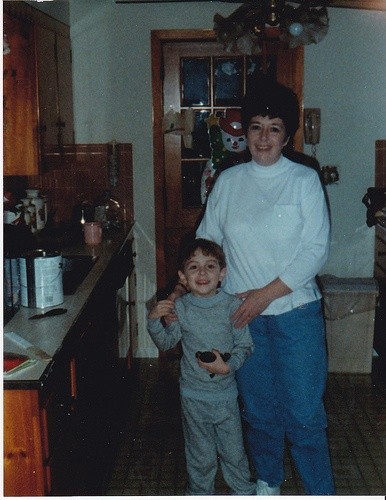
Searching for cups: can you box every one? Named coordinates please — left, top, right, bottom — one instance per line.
left=82, top=222, right=103, bottom=244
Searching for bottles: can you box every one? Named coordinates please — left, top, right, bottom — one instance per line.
left=3, top=188, right=48, bottom=235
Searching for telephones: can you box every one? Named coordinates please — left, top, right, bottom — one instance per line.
left=304, top=108, right=321, bottom=145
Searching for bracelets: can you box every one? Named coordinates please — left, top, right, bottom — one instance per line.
left=174, top=282, right=189, bottom=293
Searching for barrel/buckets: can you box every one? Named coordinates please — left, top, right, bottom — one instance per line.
left=17, top=248, right=64, bottom=310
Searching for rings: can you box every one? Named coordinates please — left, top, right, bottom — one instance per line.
left=248, top=316, right=251, bottom=320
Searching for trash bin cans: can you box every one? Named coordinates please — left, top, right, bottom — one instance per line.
left=321, top=276, right=379, bottom=375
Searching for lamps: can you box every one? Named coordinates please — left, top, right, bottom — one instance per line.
left=214, top=0, right=329, bottom=54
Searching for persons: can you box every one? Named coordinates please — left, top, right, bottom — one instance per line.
left=165, top=81, right=334, bottom=496
left=146, top=238, right=254, bottom=496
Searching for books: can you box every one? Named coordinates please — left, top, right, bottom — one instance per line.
left=4, top=331, right=53, bottom=378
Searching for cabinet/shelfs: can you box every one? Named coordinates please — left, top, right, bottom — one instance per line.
left=3, top=0, right=136, bottom=496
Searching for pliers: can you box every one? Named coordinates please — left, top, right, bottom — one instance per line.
left=30, top=308, right=67, bottom=319
